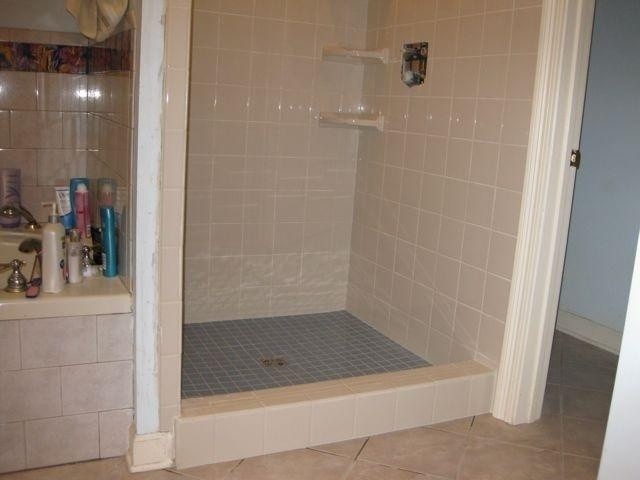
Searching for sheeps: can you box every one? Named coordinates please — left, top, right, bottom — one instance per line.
left=1, top=200, right=42, bottom=229
